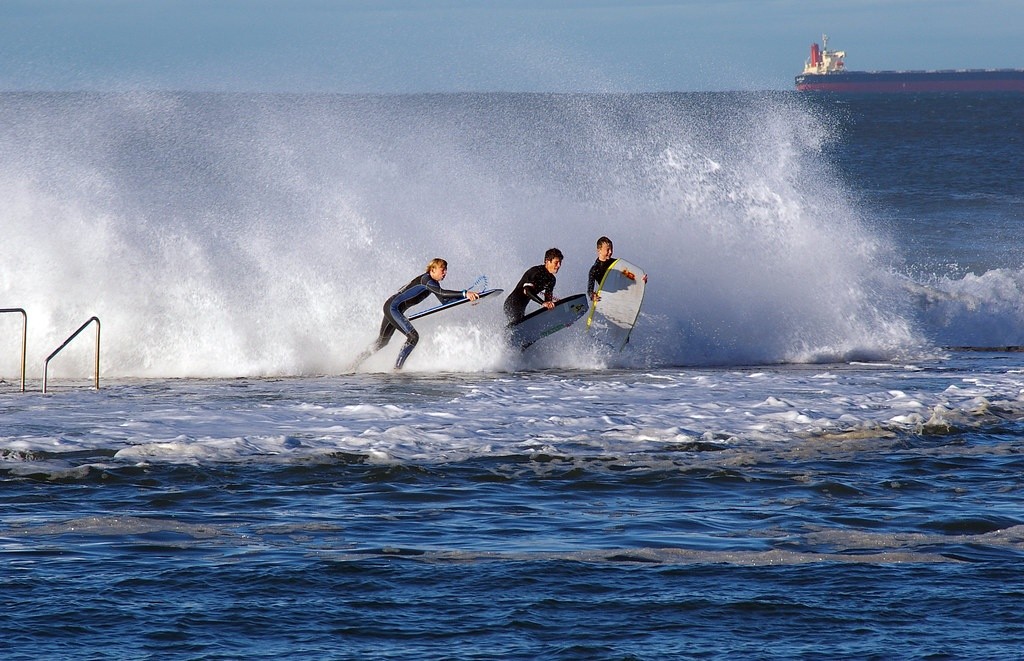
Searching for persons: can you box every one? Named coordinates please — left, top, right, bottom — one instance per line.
left=503, top=248, right=572, bottom=328
left=356, top=258, right=480, bottom=370
left=587, top=236, right=648, bottom=344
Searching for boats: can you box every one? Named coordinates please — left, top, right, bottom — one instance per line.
left=795, top=34, right=1024, bottom=93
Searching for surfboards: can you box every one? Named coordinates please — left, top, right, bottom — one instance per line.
left=407, top=288, right=505, bottom=322
left=502, top=293, right=590, bottom=351
left=584, top=257, right=647, bottom=353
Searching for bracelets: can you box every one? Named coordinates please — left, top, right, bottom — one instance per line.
left=463, top=289, right=465, bottom=298
